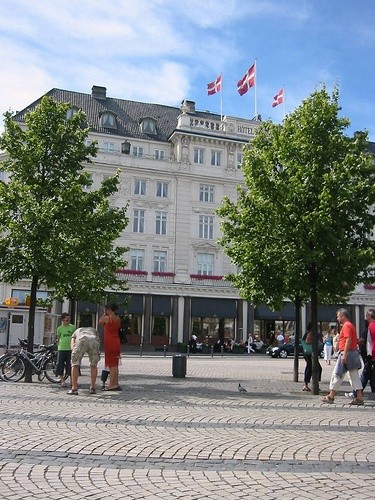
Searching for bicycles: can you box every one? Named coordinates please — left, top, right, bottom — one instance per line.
left=0, top=337, right=69, bottom=383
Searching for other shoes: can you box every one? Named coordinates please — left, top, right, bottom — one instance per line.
left=61, top=383, right=67, bottom=387
left=349, top=398, right=364, bottom=405
left=66, top=389, right=78, bottom=395
left=345, top=393, right=355, bottom=400
left=303, top=386, right=311, bottom=392
left=89, top=388, right=96, bottom=394
left=321, top=396, right=334, bottom=404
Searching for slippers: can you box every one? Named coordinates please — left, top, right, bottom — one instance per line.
left=102, top=386, right=122, bottom=391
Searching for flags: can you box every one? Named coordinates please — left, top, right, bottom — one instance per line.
left=272, top=88, right=284, bottom=108
left=207, top=75, right=221, bottom=95
left=237, top=64, right=255, bottom=96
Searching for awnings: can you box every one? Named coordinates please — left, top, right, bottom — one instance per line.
left=192, top=297, right=237, bottom=319
left=254, top=301, right=297, bottom=321
left=107, top=293, right=143, bottom=314
left=73, top=295, right=97, bottom=313
left=152, top=296, right=172, bottom=316
left=305, top=302, right=348, bottom=322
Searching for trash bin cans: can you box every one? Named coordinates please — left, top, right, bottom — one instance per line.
left=172, top=354, right=186, bottom=378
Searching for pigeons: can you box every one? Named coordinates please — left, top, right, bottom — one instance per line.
left=238, top=383, right=247, bottom=393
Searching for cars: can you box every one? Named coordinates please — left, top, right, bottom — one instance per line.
left=266, top=339, right=324, bottom=358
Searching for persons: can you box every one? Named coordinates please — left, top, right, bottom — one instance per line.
left=254, top=337, right=263, bottom=350
left=66, top=327, right=100, bottom=395
left=56, top=312, right=76, bottom=387
left=331, top=329, right=340, bottom=359
left=99, top=304, right=122, bottom=390
left=323, top=330, right=333, bottom=365
left=301, top=322, right=322, bottom=391
left=345, top=309, right=375, bottom=398
left=358, top=327, right=375, bottom=392
left=320, top=308, right=365, bottom=405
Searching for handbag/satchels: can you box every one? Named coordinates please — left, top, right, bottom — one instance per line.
left=301, top=340, right=323, bottom=356
